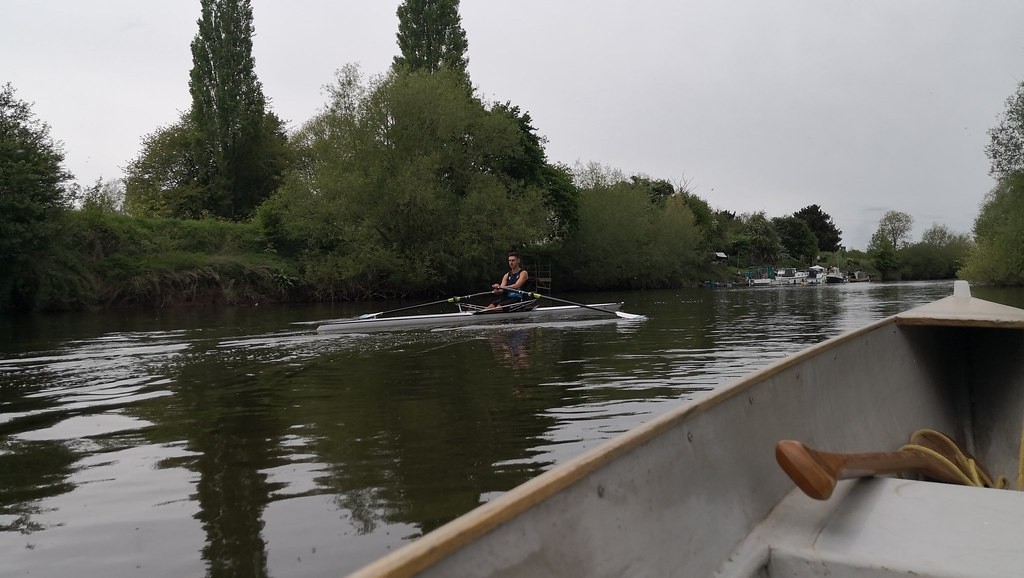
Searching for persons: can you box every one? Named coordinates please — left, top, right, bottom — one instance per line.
left=486, top=252, right=530, bottom=309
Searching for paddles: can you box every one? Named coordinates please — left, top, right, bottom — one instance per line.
left=772, top=428, right=971, bottom=502
left=290, top=287, right=503, bottom=325
left=498, top=284, right=646, bottom=320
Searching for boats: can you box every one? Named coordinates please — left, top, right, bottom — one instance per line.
left=316, top=300, right=625, bottom=337
left=746, top=264, right=872, bottom=285
